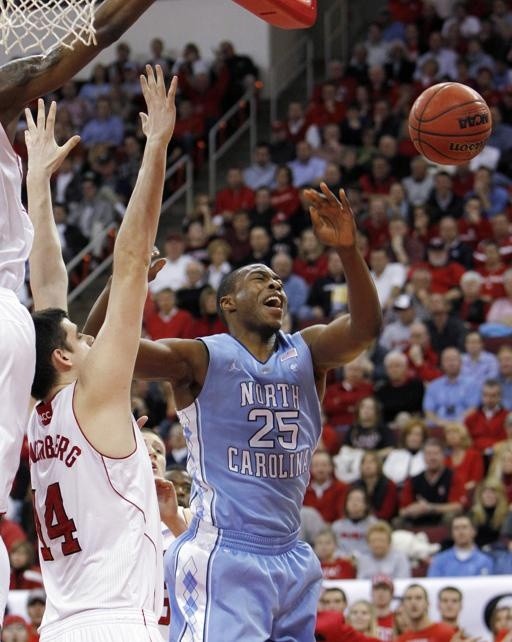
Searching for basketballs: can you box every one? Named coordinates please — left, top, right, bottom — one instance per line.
left=408, top=81, right=493, bottom=166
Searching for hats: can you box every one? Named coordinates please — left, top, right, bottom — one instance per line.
left=373, top=574, right=392, bottom=591
left=392, top=294, right=412, bottom=310
